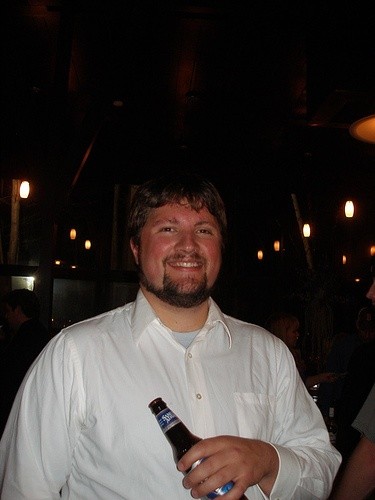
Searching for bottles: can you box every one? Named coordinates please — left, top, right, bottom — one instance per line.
left=149, top=398, right=249, bottom=500
left=327, top=408, right=336, bottom=446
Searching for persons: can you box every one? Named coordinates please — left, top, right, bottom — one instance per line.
left=325, top=385, right=375, bottom=500
left=0, top=173, right=342, bottom=500
left=0, top=288, right=55, bottom=440
left=272, top=317, right=338, bottom=390
